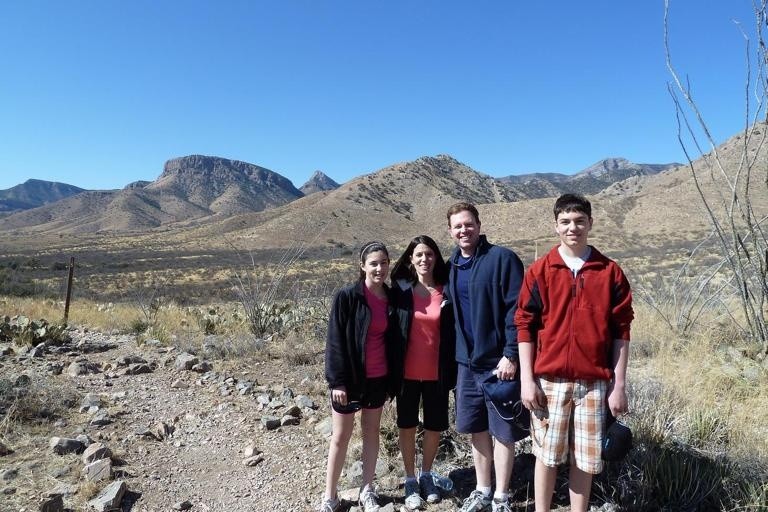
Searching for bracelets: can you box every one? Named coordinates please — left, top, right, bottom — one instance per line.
left=505, top=355, right=516, bottom=361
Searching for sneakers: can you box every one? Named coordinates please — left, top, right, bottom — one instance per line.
left=357, top=486, right=380, bottom=512
left=404, top=476, right=426, bottom=510
left=319, top=492, right=341, bottom=512
left=457, top=489, right=493, bottom=512
left=491, top=497, right=513, bottom=512
left=416, top=469, right=441, bottom=504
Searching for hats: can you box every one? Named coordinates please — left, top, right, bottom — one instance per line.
left=480, top=368, right=525, bottom=422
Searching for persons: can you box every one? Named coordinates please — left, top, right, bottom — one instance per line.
left=324, top=241, right=393, bottom=511
left=443, top=203, right=527, bottom=511
left=514, top=192, right=634, bottom=510
left=384, top=235, right=448, bottom=510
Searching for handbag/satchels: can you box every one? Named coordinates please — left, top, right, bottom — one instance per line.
left=601, top=416, right=635, bottom=463
